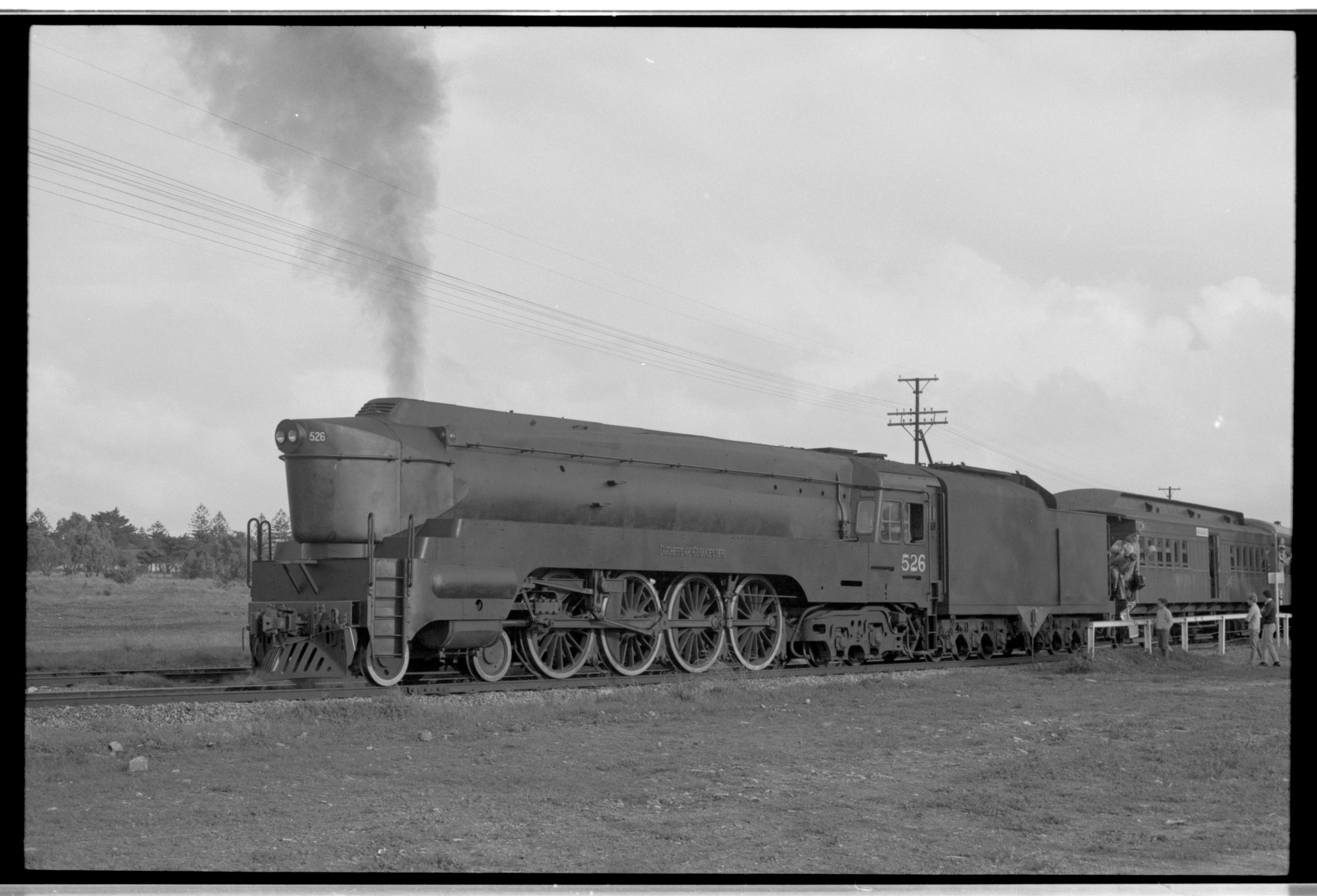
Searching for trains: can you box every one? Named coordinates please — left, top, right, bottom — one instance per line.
left=243, top=396, right=1290, bottom=700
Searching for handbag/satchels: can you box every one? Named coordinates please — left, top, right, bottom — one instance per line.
left=1132, top=575, right=1145, bottom=590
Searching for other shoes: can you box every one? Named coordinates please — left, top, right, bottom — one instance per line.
left=1273, top=662, right=1281, bottom=667
left=1111, top=591, right=1117, bottom=599
left=1259, top=662, right=1268, bottom=666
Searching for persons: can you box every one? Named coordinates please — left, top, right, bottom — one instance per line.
left=1278, top=545, right=1289, bottom=600
left=1107, top=530, right=1140, bottom=608
left=1245, top=593, right=1262, bottom=664
left=1154, top=598, right=1174, bottom=657
left=1259, top=589, right=1281, bottom=668
